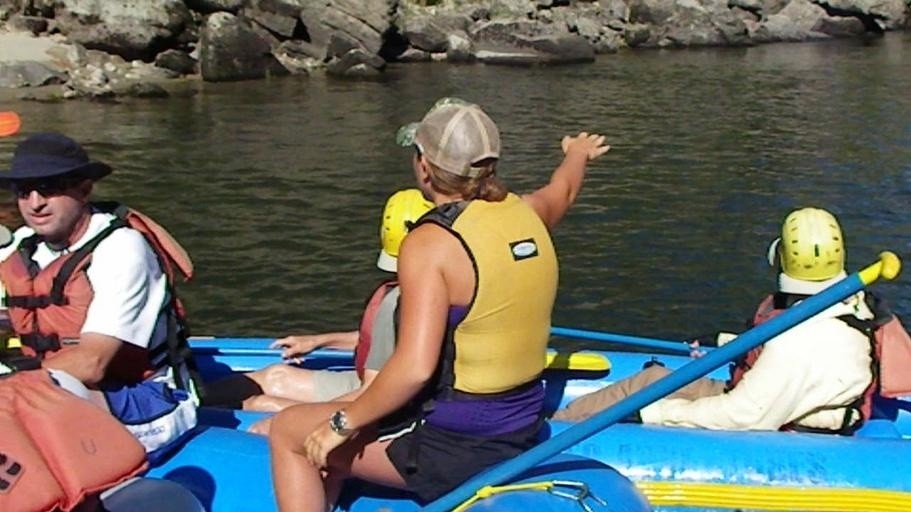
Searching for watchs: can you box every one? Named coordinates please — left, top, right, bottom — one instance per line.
left=326, top=407, right=354, bottom=438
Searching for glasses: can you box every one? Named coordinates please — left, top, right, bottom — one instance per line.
left=9, top=177, right=71, bottom=198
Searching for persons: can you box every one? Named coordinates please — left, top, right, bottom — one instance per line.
left=268, top=96, right=612, bottom=512
left=550, top=206, right=877, bottom=435
left=1, top=131, right=207, bottom=462
left=241, top=191, right=435, bottom=434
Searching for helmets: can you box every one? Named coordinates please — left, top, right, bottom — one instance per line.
left=779, top=208, right=845, bottom=281
left=381, top=189, right=434, bottom=257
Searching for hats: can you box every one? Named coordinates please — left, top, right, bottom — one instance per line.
left=397, top=98, right=501, bottom=178
left=0, top=133, right=112, bottom=190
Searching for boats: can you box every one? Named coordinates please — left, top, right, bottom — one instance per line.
left=0, top=429, right=653, bottom=512
left=188, top=337, right=910, bottom=511
